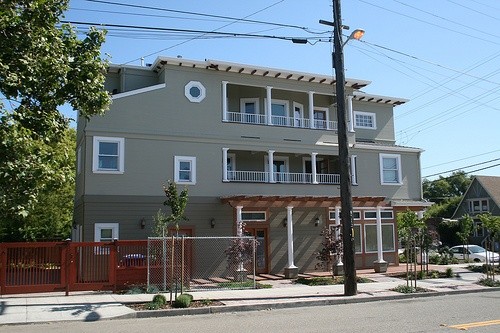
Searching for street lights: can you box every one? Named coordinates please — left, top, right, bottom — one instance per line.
left=334, top=28, right=366, bottom=296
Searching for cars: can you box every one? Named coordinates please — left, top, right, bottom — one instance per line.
left=403, top=247, right=429, bottom=265
left=441, top=244, right=500, bottom=264
